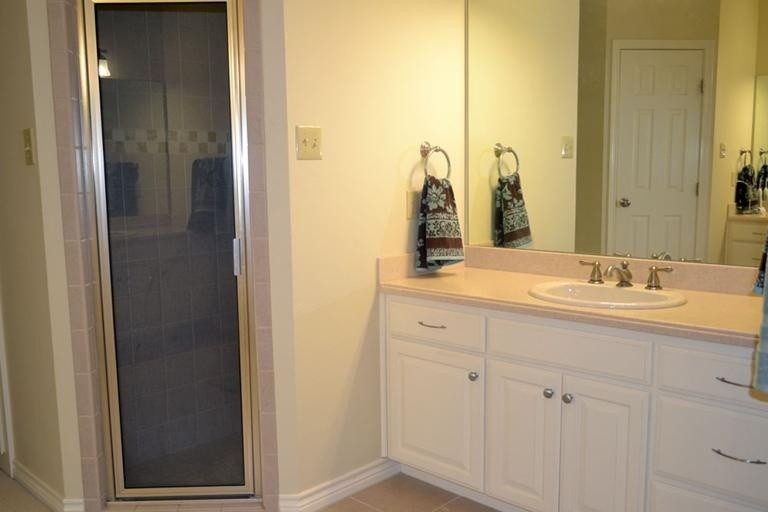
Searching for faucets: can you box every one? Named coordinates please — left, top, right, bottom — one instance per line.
left=651, top=250, right=673, bottom=260
left=604, top=259, right=635, bottom=288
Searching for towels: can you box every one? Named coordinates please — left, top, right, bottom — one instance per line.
left=735, top=165, right=768, bottom=214
left=493, top=173, right=533, bottom=250
left=413, top=175, right=465, bottom=276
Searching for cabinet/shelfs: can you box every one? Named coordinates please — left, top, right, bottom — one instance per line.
left=724, top=219, right=768, bottom=268
left=484, top=310, right=654, bottom=511
left=651, top=334, right=768, bottom=512
left=378, top=294, right=488, bottom=504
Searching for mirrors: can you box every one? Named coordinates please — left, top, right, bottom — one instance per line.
left=100, top=78, right=174, bottom=230
left=461, top=0, right=768, bottom=269
left=750, top=73, right=768, bottom=202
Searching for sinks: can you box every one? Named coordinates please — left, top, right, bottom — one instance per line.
left=529, top=279, right=688, bottom=309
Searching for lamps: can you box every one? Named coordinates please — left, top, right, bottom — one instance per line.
left=97, top=46, right=111, bottom=77
